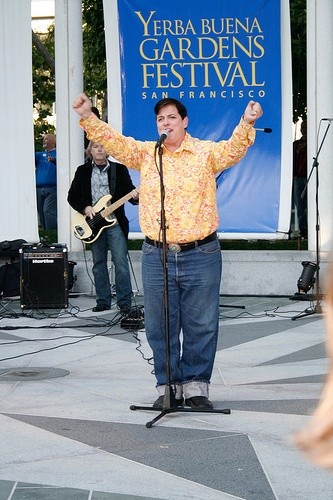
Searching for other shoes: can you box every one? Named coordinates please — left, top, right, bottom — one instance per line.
left=92, top=306, right=105, bottom=312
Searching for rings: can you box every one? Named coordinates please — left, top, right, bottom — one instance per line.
left=255, top=111, right=258, bottom=114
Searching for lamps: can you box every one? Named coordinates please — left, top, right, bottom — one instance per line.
left=68, top=261, right=79, bottom=293
left=289, top=261, right=327, bottom=301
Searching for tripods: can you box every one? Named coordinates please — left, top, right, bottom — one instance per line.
left=130, top=149, right=230, bottom=426
left=289, top=122, right=325, bottom=322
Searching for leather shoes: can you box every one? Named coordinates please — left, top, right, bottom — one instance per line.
left=185, top=396, right=213, bottom=409
left=153, top=395, right=163, bottom=407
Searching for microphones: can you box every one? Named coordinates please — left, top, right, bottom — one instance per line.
left=155, top=130, right=169, bottom=148
left=322, top=117, right=333, bottom=122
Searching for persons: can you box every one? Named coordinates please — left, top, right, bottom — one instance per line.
left=294, top=265, right=333, bottom=469
left=72, top=94, right=263, bottom=409
left=36, top=108, right=140, bottom=312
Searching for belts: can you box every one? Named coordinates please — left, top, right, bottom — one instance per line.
left=144, top=232, right=217, bottom=253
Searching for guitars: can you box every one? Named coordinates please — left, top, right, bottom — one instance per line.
left=72, top=189, right=139, bottom=244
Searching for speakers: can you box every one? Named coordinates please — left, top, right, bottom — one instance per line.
left=19, top=243, right=68, bottom=309
